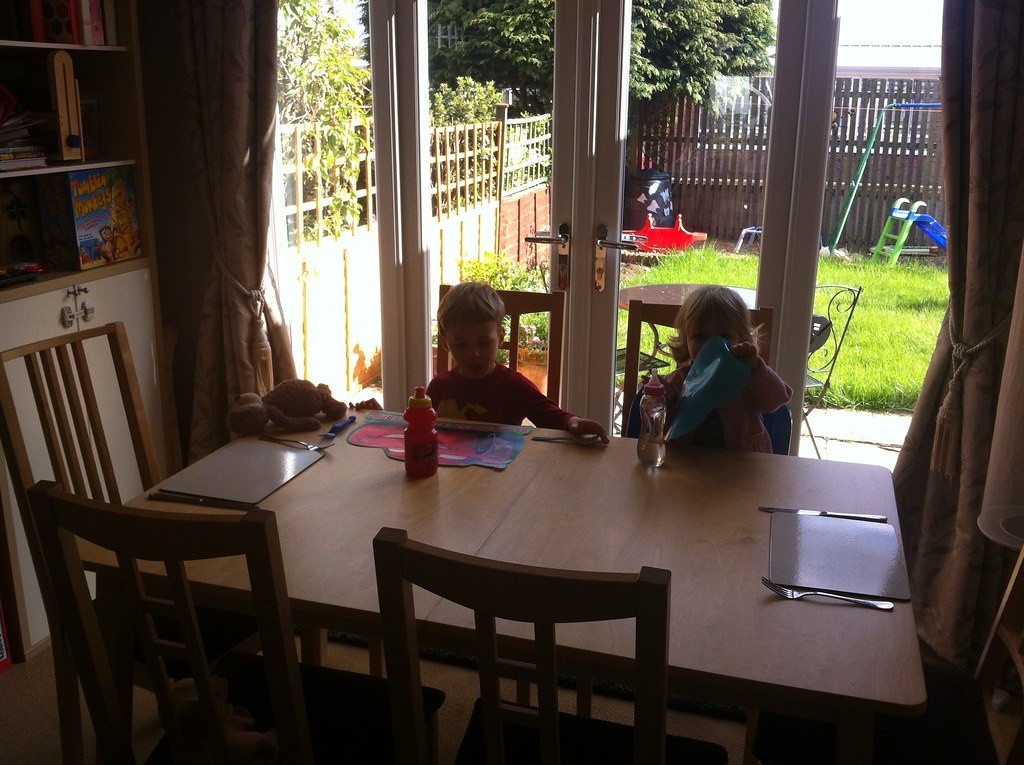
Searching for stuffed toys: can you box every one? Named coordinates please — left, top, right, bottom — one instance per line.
left=227, top=379, right=346, bottom=430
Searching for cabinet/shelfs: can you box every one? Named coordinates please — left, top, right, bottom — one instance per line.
left=0, top=1, right=184, bottom=665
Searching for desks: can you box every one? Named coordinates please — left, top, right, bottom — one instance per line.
left=78, top=406, right=927, bottom=765
left=613, top=283, right=759, bottom=425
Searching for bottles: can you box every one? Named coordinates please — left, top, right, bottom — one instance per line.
left=403, top=386, right=438, bottom=477
left=637, top=369, right=666, bottom=468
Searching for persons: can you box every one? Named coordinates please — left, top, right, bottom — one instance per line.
left=641, top=285, right=793, bottom=454
left=424, top=283, right=610, bottom=443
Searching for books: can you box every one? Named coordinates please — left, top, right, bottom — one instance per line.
left=0, top=111, right=47, bottom=171
left=17, top=0, right=117, bottom=46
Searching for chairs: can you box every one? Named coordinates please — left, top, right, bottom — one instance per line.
left=743, top=539, right=1024, bottom=765
left=25, top=478, right=443, bottom=765
left=437, top=284, right=565, bottom=404
left=373, top=527, right=730, bottom=765
left=802, top=283, right=864, bottom=461
left=620, top=299, right=774, bottom=449
left=0, top=322, right=327, bottom=765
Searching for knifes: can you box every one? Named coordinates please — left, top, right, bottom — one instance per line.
left=149, top=493, right=259, bottom=511
left=757, top=506, right=888, bottom=522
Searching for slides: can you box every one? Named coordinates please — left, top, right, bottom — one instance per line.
left=890, top=207, right=947, bottom=251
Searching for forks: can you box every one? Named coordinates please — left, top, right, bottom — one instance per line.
left=257, top=434, right=335, bottom=451
left=319, top=416, right=356, bottom=437
left=532, top=435, right=600, bottom=445
left=761, top=575, right=894, bottom=609
